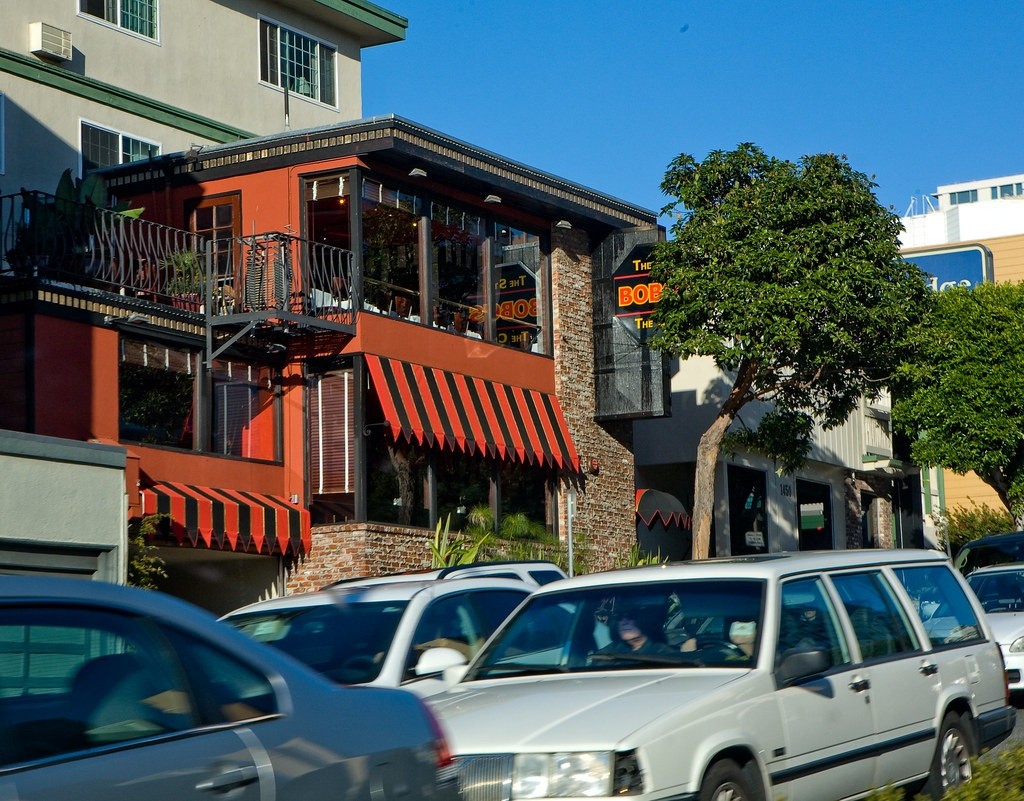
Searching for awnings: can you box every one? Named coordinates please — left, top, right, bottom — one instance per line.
left=364, top=352, right=580, bottom=473
left=139, top=480, right=311, bottom=559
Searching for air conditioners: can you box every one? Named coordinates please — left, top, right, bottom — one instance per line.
left=30, top=21, right=73, bottom=63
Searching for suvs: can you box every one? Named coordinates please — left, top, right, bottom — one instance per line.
left=418, top=548, right=1018, bottom=801
left=952, top=533, right=1024, bottom=578
left=317, top=560, right=571, bottom=592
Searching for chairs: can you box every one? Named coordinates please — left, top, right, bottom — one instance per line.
left=71, top=654, right=172, bottom=740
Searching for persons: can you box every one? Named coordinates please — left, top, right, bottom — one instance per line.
left=683, top=615, right=757, bottom=662
left=588, top=606, right=682, bottom=666
left=802, top=606, right=822, bottom=627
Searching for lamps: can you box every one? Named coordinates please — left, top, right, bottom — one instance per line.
left=408, top=168, right=428, bottom=179
left=483, top=194, right=502, bottom=203
left=555, top=220, right=572, bottom=230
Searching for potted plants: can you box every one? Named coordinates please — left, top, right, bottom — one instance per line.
left=159, top=245, right=215, bottom=313
left=4, top=224, right=38, bottom=278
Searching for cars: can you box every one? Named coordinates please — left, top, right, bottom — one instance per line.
left=211, top=576, right=614, bottom=703
left=0, top=573, right=462, bottom=801
left=964, top=561, right=1024, bottom=690
